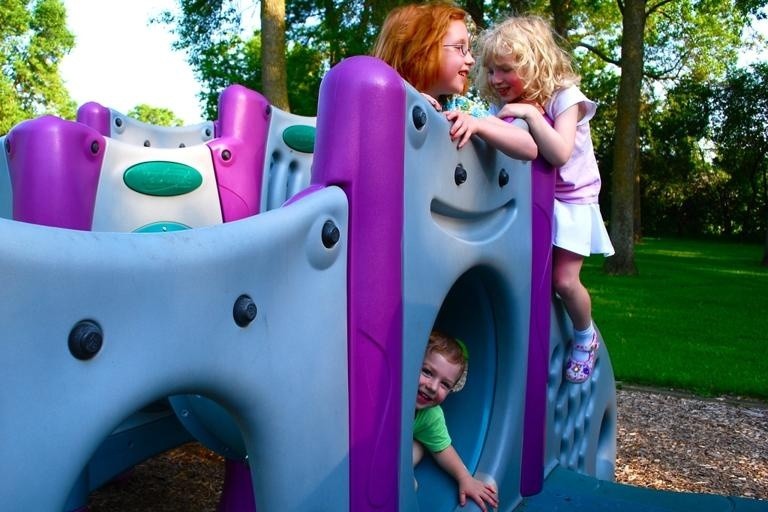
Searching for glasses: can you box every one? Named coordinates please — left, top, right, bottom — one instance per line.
left=443, top=38, right=472, bottom=56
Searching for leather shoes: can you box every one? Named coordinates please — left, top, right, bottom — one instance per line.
left=564, top=330, right=600, bottom=382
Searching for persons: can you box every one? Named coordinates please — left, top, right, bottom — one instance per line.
left=475, top=12, right=617, bottom=385
left=409, top=331, right=504, bottom=512
left=367, top=1, right=540, bottom=162
